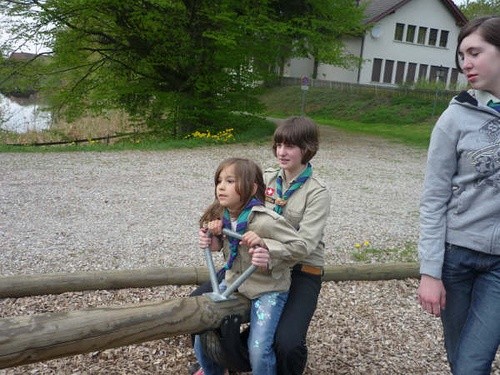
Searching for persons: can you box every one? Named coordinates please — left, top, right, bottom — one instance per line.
left=418, top=15, right=500, bottom=375
left=189, top=116, right=330, bottom=375
left=194, top=157, right=309, bottom=375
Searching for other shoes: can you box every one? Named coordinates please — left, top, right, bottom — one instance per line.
left=192, top=366, right=229, bottom=375
